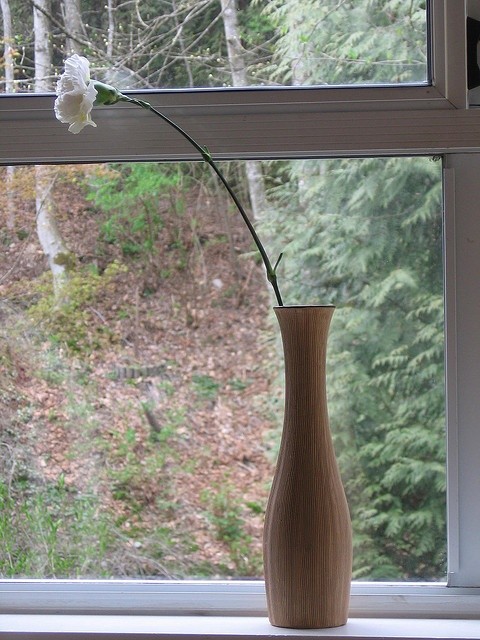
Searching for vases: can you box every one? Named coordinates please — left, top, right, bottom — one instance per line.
left=263, top=306, right=353, bottom=628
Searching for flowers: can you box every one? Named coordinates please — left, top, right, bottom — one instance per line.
left=55, top=54, right=283, bottom=306
